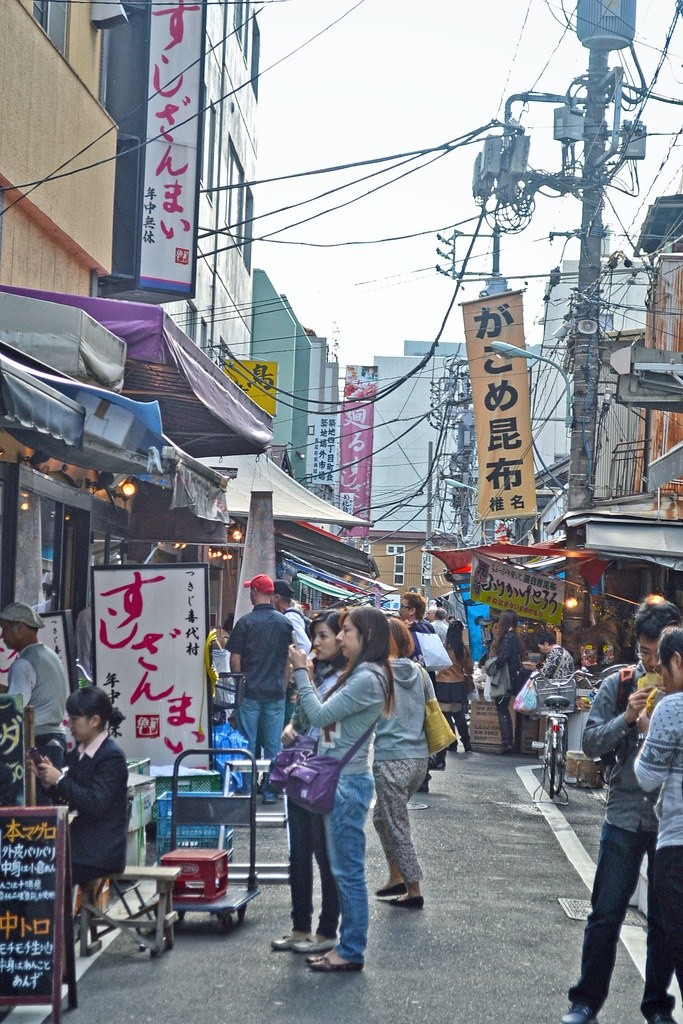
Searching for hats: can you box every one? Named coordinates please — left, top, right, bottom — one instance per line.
left=0, top=600, right=47, bottom=630
left=243, top=574, right=275, bottom=595
left=273, top=578, right=296, bottom=599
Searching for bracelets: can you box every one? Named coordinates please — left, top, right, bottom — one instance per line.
left=293, top=668, right=308, bottom=673
left=639, top=732, right=647, bottom=741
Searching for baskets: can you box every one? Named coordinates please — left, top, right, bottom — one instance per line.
left=535, top=678, right=577, bottom=716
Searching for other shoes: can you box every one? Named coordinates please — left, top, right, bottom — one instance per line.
left=309, top=954, right=364, bottom=973
left=375, top=882, right=424, bottom=909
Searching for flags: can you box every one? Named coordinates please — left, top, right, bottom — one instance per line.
left=460, top=288, right=540, bottom=517
left=468, top=552, right=567, bottom=626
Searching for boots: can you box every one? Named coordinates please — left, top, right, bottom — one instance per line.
left=494, top=711, right=518, bottom=756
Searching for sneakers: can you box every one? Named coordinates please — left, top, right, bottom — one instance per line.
left=262, top=790, right=279, bottom=805
left=422, top=698, right=459, bottom=756
left=560, top=1001, right=599, bottom=1024
left=646, top=1011, right=680, bottom=1024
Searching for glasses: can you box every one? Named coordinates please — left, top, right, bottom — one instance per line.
left=401, top=604, right=411, bottom=610
left=634, top=645, right=660, bottom=660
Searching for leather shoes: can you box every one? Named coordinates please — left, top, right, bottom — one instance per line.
left=268, top=930, right=313, bottom=949
left=293, top=933, right=339, bottom=954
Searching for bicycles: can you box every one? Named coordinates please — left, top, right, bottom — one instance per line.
left=518, top=667, right=593, bottom=805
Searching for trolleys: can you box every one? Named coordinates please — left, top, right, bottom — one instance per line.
left=140, top=748, right=260, bottom=934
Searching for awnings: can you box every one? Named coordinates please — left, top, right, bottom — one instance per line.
left=192, top=455, right=373, bottom=527
left=0, top=282, right=273, bottom=455
left=231, top=515, right=399, bottom=606
left=163, top=428, right=232, bottom=525
left=1, top=355, right=171, bottom=473
left=1, top=358, right=87, bottom=453
left=434, top=508, right=683, bottom=600
left=0, top=289, right=128, bottom=394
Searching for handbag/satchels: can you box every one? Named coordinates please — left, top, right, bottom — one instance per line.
left=281, top=752, right=342, bottom=815
left=463, top=673, right=476, bottom=694
left=513, top=678, right=538, bottom=716
left=268, top=735, right=316, bottom=790
left=409, top=619, right=454, bottom=674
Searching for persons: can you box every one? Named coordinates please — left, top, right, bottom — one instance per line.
left=25, top=687, right=128, bottom=888
left=288, top=594, right=396, bottom=973
left=426, top=607, right=455, bottom=643
left=400, top=593, right=454, bottom=792
left=2, top=603, right=67, bottom=764
left=562, top=603, right=681, bottom=1024
left=270, top=611, right=350, bottom=952
left=479, top=610, right=575, bottom=754
left=229, top=575, right=293, bottom=803
left=271, top=578, right=312, bottom=728
left=437, top=621, right=474, bottom=752
left=375, top=616, right=438, bottom=908
left=634, top=626, right=683, bottom=1003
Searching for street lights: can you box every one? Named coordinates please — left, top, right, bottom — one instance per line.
left=487, top=339, right=603, bottom=670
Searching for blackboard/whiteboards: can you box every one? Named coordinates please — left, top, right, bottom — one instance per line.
left=3, top=806, right=73, bottom=1004
left=1, top=693, right=25, bottom=807
